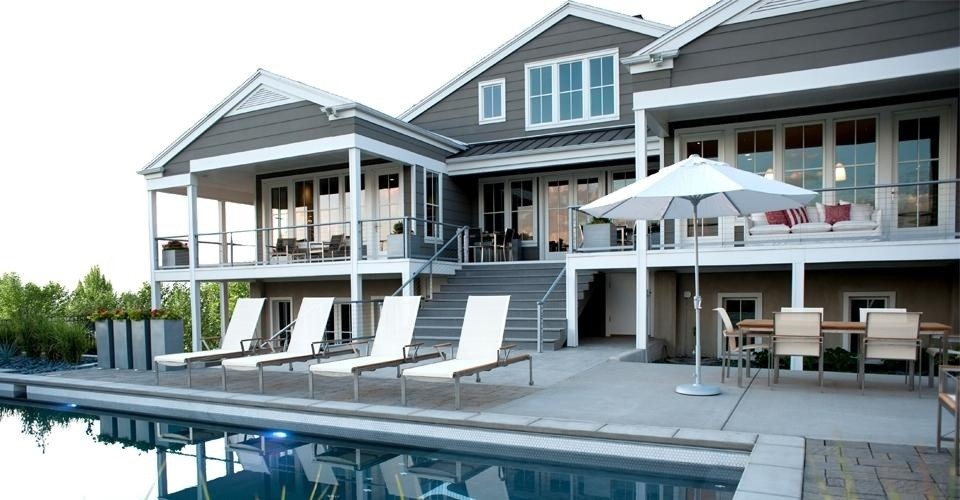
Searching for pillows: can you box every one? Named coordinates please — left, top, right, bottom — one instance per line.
left=765, top=209, right=792, bottom=226
left=816, top=203, right=827, bottom=222
left=787, top=207, right=808, bottom=224
left=825, top=206, right=851, bottom=224
left=840, top=201, right=873, bottom=221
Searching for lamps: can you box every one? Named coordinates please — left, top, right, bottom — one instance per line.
left=835, top=144, right=846, bottom=181
left=764, top=152, right=773, bottom=179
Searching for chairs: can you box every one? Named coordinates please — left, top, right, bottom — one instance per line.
left=221, top=297, right=360, bottom=393
left=712, top=307, right=960, bottom=398
left=153, top=298, right=284, bottom=388
left=468, top=227, right=515, bottom=261
left=155, top=422, right=508, bottom=500
left=309, top=234, right=343, bottom=263
left=936, top=334, right=959, bottom=474
left=308, top=296, right=446, bottom=400
left=266, top=239, right=296, bottom=263
left=401, top=295, right=533, bottom=410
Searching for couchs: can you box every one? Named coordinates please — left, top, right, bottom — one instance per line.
left=749, top=203, right=883, bottom=245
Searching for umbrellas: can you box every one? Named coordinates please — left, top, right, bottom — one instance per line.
left=578, top=154, right=818, bottom=384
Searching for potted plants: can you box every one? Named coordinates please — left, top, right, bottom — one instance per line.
left=583, top=216, right=617, bottom=253
left=162, top=240, right=189, bottom=269
left=394, top=222, right=404, bottom=234
